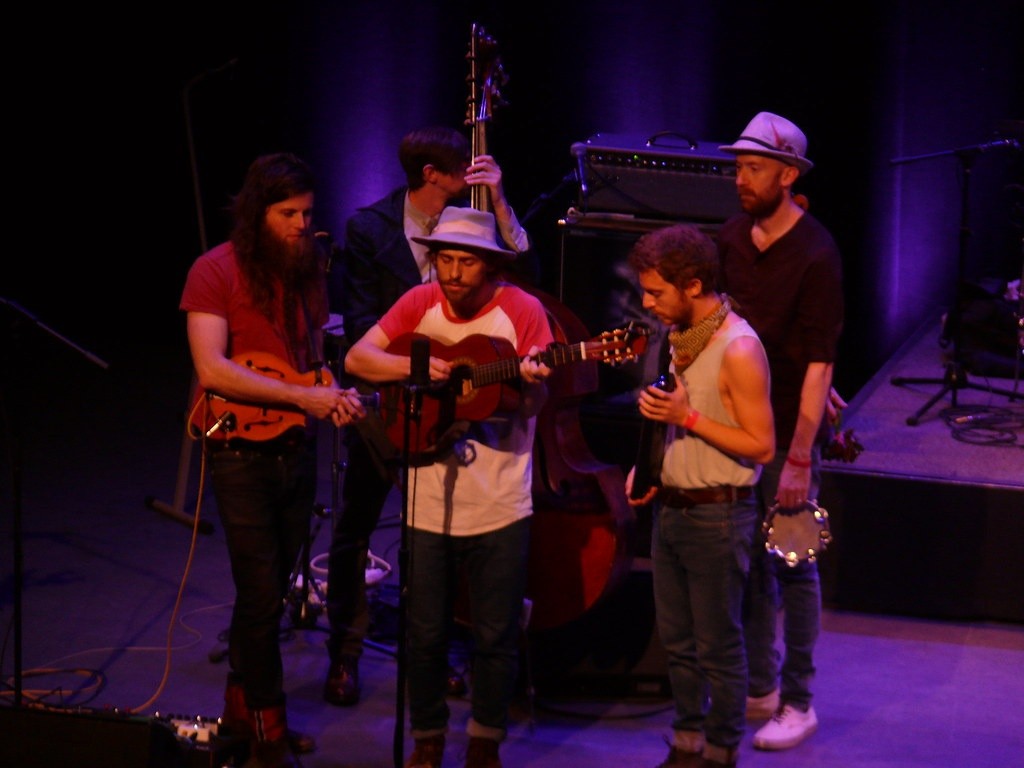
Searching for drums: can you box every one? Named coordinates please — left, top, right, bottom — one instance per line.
left=761, top=497, right=832, bottom=567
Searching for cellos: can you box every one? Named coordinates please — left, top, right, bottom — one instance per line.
left=450, top=24, right=627, bottom=634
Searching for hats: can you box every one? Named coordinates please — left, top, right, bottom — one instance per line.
left=717, top=110, right=815, bottom=175
left=410, top=205, right=518, bottom=267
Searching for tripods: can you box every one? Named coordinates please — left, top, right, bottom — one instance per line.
left=208, top=524, right=399, bottom=662
left=889, top=138, right=1024, bottom=425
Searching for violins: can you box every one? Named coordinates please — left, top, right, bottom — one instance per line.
left=630, top=373, right=676, bottom=498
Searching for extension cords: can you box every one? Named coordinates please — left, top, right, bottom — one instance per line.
left=320, top=569, right=383, bottom=595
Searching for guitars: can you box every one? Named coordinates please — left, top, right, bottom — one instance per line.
left=374, top=324, right=652, bottom=457
left=209, top=352, right=379, bottom=441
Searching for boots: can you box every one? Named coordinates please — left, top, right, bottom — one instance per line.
left=251, top=696, right=317, bottom=755
left=220, top=675, right=261, bottom=740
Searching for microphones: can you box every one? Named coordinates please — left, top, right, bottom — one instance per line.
left=411, top=338, right=430, bottom=427
left=979, top=139, right=1021, bottom=153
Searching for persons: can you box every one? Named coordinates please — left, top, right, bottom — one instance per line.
left=180, top=153, right=370, bottom=768
left=345, top=206, right=555, bottom=768
left=711, top=112, right=845, bottom=750
left=320, top=126, right=530, bottom=705
left=625, top=225, right=775, bottom=768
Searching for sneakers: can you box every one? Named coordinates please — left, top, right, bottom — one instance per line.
left=745, top=688, right=780, bottom=721
left=751, top=705, right=819, bottom=751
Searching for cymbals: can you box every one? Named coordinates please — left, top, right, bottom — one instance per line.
left=763, top=508, right=834, bottom=568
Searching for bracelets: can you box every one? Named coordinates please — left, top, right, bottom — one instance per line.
left=683, top=408, right=700, bottom=427
left=786, top=456, right=812, bottom=468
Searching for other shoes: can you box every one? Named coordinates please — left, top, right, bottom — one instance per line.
left=464, top=718, right=505, bottom=768
left=324, top=650, right=360, bottom=706
left=404, top=726, right=449, bottom=768
left=654, top=745, right=737, bottom=768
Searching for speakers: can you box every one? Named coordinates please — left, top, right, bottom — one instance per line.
left=554, top=218, right=721, bottom=417
left=0, top=701, right=204, bottom=768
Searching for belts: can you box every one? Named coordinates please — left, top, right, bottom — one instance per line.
left=657, top=484, right=755, bottom=510
